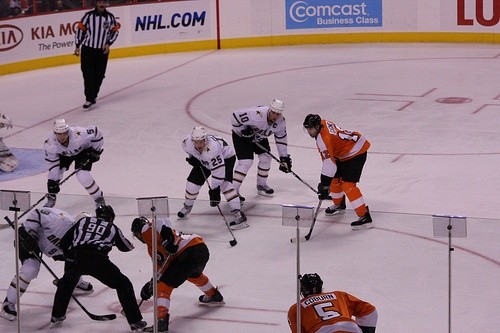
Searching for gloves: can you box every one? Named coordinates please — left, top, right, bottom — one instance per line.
left=208, top=185, right=221, bottom=207
left=186, top=156, right=203, bottom=170
left=317, top=183, right=329, bottom=200
left=162, top=239, right=179, bottom=256
left=21, top=236, right=38, bottom=251
left=48, top=179, right=61, bottom=193
left=241, top=125, right=255, bottom=143
left=279, top=154, right=293, bottom=173
left=141, top=282, right=153, bottom=300
left=63, top=248, right=75, bottom=263
left=86, top=147, right=104, bottom=163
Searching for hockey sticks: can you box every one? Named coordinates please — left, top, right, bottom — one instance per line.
left=120, top=231, right=184, bottom=318
left=4, top=215, right=117, bottom=321
left=252, top=139, right=318, bottom=193
left=12, top=159, right=90, bottom=223
left=289, top=198, right=323, bottom=243
left=199, top=165, right=237, bottom=247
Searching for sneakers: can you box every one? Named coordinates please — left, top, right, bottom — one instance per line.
left=177, top=181, right=374, bottom=230
left=95, top=191, right=106, bottom=208
left=82, top=98, right=96, bottom=111
left=0, top=280, right=225, bottom=333
left=43, top=195, right=56, bottom=208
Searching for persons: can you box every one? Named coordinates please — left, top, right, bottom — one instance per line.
left=231, top=100, right=293, bottom=206
left=131, top=217, right=225, bottom=333
left=177, top=126, right=249, bottom=230
left=43, top=119, right=106, bottom=209
left=75, top=0, right=120, bottom=112
left=0, top=207, right=94, bottom=321
left=302, top=113, right=375, bottom=231
left=0, top=114, right=19, bottom=173
left=48, top=205, right=148, bottom=333
left=287, top=272, right=378, bottom=333
left=0, top=0, right=131, bottom=19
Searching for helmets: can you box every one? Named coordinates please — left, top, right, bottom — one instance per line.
left=53, top=118, right=69, bottom=133
left=303, top=114, right=321, bottom=130
left=95, top=205, right=115, bottom=219
left=300, top=272, right=323, bottom=295
left=74, top=211, right=91, bottom=225
left=268, top=99, right=283, bottom=116
left=132, top=216, right=149, bottom=232
left=191, top=126, right=208, bottom=145
